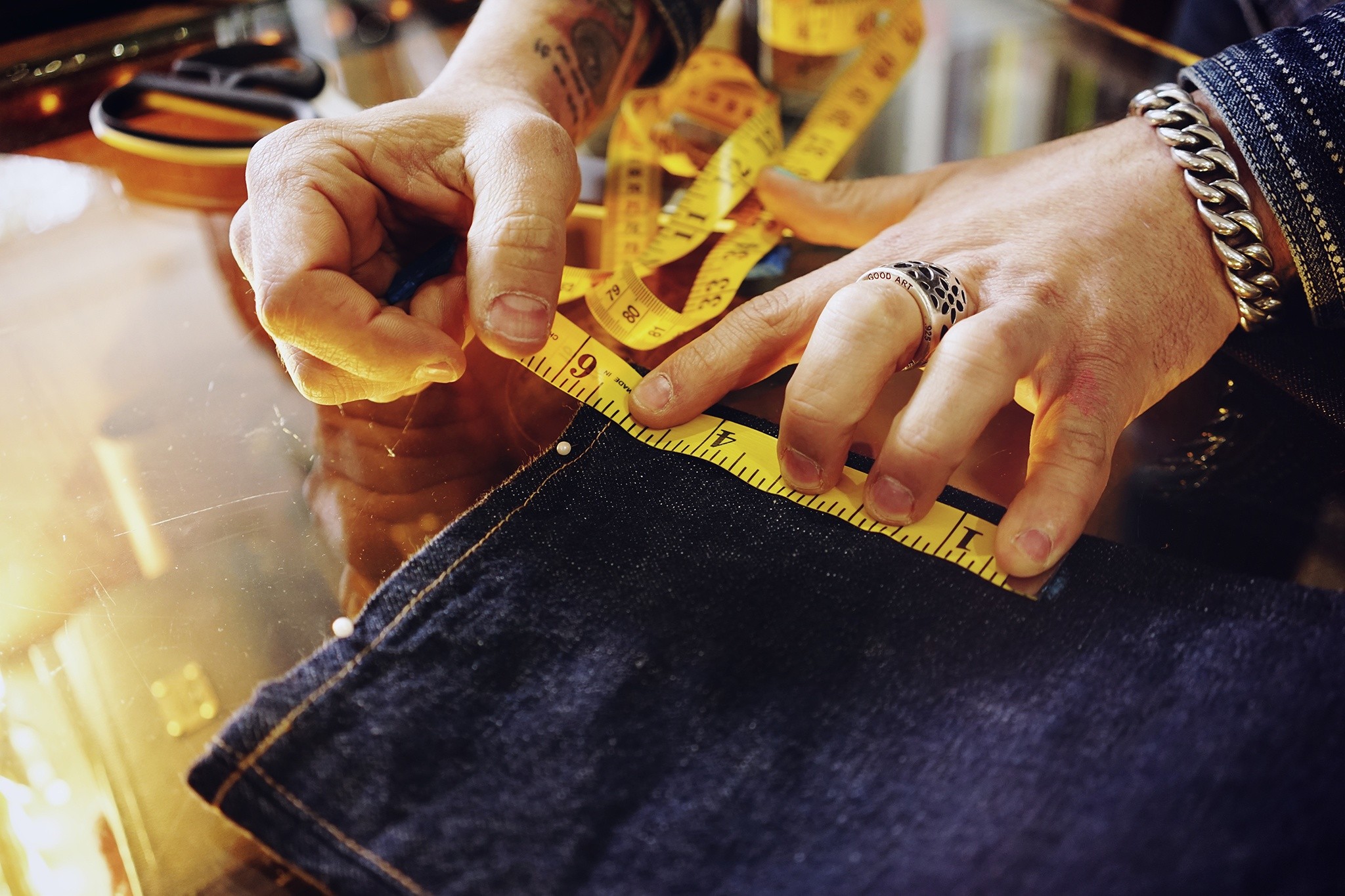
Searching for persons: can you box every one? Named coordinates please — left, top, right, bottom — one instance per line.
left=228, top=0, right=1345, bottom=579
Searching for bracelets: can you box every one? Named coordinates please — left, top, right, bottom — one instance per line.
left=1130, top=83, right=1283, bottom=332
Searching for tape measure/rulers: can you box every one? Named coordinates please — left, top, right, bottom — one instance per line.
left=502, top=2, right=1063, bottom=599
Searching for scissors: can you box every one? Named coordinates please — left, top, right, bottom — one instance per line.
left=90, top=41, right=327, bottom=174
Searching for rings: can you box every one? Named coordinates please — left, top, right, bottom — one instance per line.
left=855, top=258, right=967, bottom=371
